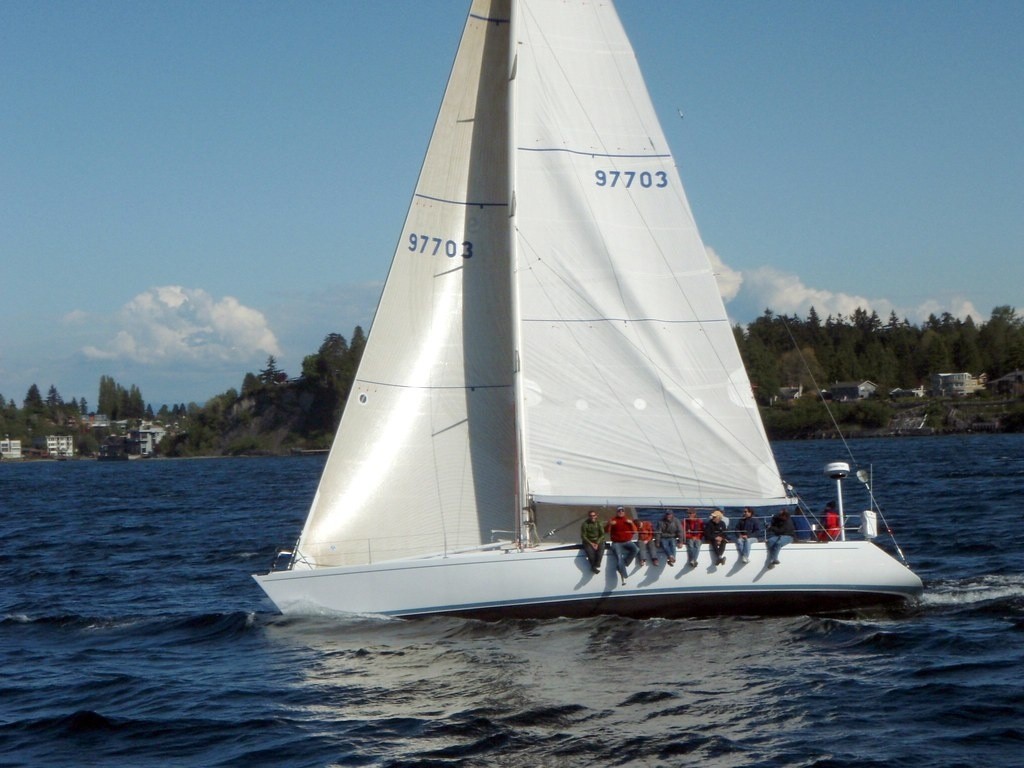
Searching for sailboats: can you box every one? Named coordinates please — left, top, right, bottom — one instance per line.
left=251, top=3, right=925, bottom=625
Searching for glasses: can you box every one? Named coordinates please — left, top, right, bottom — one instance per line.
left=618, top=510, right=624, bottom=512
left=745, top=511, right=747, bottom=513
left=589, top=515, right=596, bottom=517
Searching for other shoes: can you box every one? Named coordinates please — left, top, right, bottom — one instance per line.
left=722, top=557, right=726, bottom=565
left=712, top=560, right=719, bottom=565
left=690, top=560, right=698, bottom=567
left=622, top=578, right=626, bottom=585
left=640, top=560, right=644, bottom=566
left=669, top=555, right=675, bottom=563
left=653, top=560, right=659, bottom=566
left=742, top=558, right=751, bottom=564
left=770, top=559, right=779, bottom=564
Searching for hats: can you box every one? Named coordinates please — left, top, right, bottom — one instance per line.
left=617, top=506, right=624, bottom=511
left=665, top=509, right=673, bottom=514
left=717, top=506, right=724, bottom=511
left=711, top=511, right=723, bottom=518
left=687, top=508, right=696, bottom=513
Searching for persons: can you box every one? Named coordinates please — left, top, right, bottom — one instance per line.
left=816, top=501, right=842, bottom=543
left=734, top=506, right=762, bottom=563
left=655, top=510, right=683, bottom=567
left=765, top=507, right=791, bottom=569
left=681, top=508, right=704, bottom=568
left=702, top=510, right=728, bottom=566
left=604, top=506, right=640, bottom=577
left=580, top=510, right=607, bottom=574
left=790, top=506, right=817, bottom=543
left=633, top=518, right=660, bottom=566
